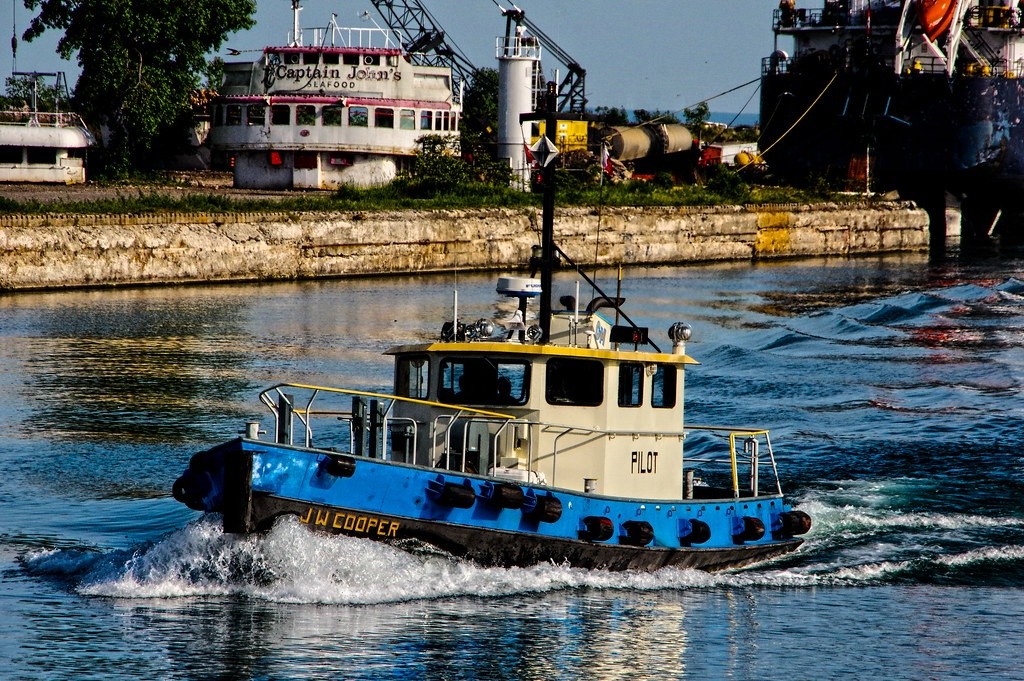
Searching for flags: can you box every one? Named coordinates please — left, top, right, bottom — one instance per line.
left=524, top=143, right=535, bottom=166
left=602, top=145, right=614, bottom=177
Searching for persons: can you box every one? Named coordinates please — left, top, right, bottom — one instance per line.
left=454, top=376, right=517, bottom=405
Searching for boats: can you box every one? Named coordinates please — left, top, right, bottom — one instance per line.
left=174, top=67, right=811, bottom=568
left=758, top=0, right=1024, bottom=237
